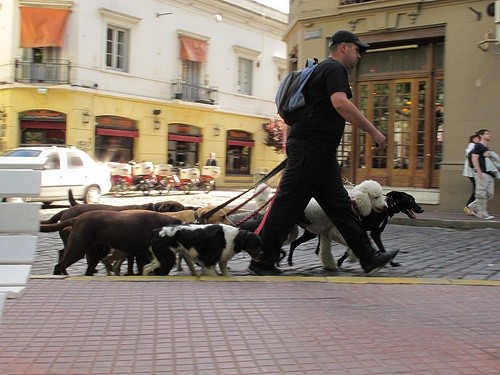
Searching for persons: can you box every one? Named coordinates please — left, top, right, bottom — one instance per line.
left=205, top=153, right=217, bottom=190
left=247, top=31, right=401, bottom=275
left=462, top=130, right=483, bottom=216
left=466, top=129, right=496, bottom=220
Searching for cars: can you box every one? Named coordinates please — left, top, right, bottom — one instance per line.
left=0, top=145, right=112, bottom=207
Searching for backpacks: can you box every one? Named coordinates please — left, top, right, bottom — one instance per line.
left=275, top=58, right=348, bottom=126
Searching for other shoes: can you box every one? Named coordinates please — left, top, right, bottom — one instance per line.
left=248, top=260, right=280, bottom=276
left=464, top=207, right=477, bottom=216
left=483, top=215, right=494, bottom=219
left=362, top=248, right=399, bottom=276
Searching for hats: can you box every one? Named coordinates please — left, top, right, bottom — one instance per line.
left=328, top=30, right=370, bottom=52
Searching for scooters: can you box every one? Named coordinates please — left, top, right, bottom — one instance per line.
left=192, top=162, right=222, bottom=195
left=153, top=163, right=180, bottom=196
left=127, top=160, right=158, bottom=196
left=172, top=162, right=201, bottom=195
left=102, top=160, right=134, bottom=198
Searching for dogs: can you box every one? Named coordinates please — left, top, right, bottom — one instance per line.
left=39, top=180, right=389, bottom=277
left=288, top=191, right=424, bottom=270
